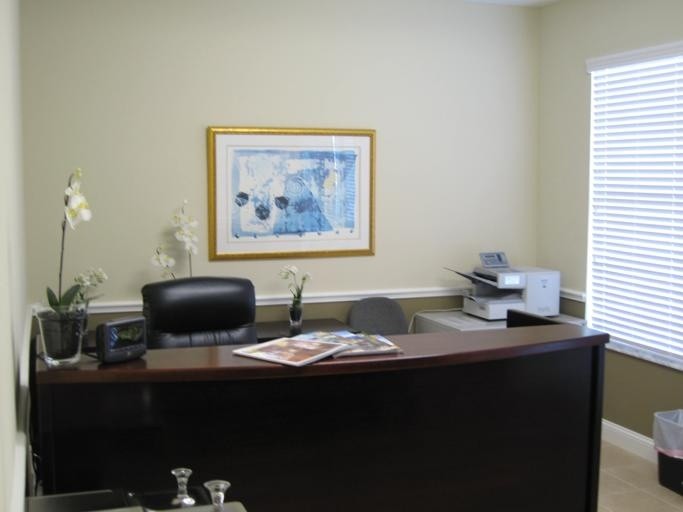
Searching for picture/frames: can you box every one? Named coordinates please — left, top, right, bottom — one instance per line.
left=207, top=126, right=377, bottom=262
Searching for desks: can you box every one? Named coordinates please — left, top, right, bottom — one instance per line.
left=256, top=318, right=360, bottom=342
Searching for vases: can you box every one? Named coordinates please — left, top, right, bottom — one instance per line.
left=288, top=296, right=304, bottom=325
left=35, top=310, right=87, bottom=367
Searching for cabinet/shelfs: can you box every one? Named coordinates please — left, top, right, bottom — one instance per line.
left=34, top=310, right=610, bottom=512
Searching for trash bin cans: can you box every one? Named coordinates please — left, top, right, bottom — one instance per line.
left=653, top=409, right=683, bottom=497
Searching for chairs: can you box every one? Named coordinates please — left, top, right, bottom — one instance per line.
left=140, top=278, right=257, bottom=348
left=349, top=297, right=409, bottom=335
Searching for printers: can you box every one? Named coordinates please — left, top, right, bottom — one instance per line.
left=444, top=252, right=560, bottom=320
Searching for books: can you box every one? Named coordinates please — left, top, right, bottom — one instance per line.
left=233, top=329, right=403, bottom=368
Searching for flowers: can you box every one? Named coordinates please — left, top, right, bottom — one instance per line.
left=154, top=198, right=201, bottom=278
left=72, top=269, right=108, bottom=310
left=40, top=168, right=95, bottom=310
left=278, top=264, right=312, bottom=295
